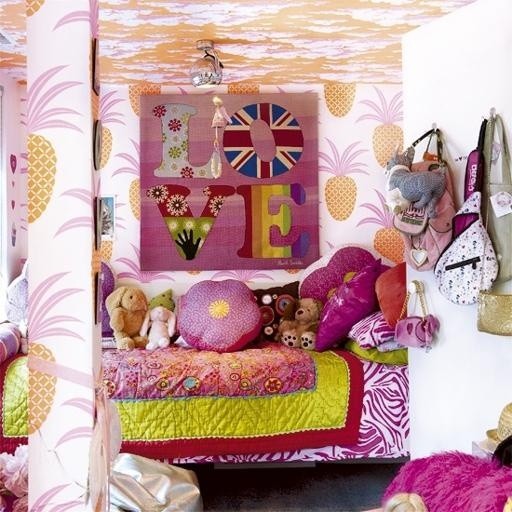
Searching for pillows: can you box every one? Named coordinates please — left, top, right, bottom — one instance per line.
left=177, top=243, right=407, bottom=365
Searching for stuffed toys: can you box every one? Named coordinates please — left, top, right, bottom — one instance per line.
left=384, top=144, right=446, bottom=218
left=381, top=449, right=512, bottom=512
left=105, top=286, right=176, bottom=351
left=278, top=298, right=324, bottom=350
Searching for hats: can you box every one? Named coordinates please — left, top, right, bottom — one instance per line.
left=486, top=402, right=512, bottom=444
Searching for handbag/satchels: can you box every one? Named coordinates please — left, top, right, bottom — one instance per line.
left=393, top=128, right=511, bottom=348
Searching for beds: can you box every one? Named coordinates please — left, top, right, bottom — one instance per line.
left=0, top=340, right=408, bottom=465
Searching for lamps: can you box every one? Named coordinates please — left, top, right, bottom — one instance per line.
left=191, top=40, right=224, bottom=88
